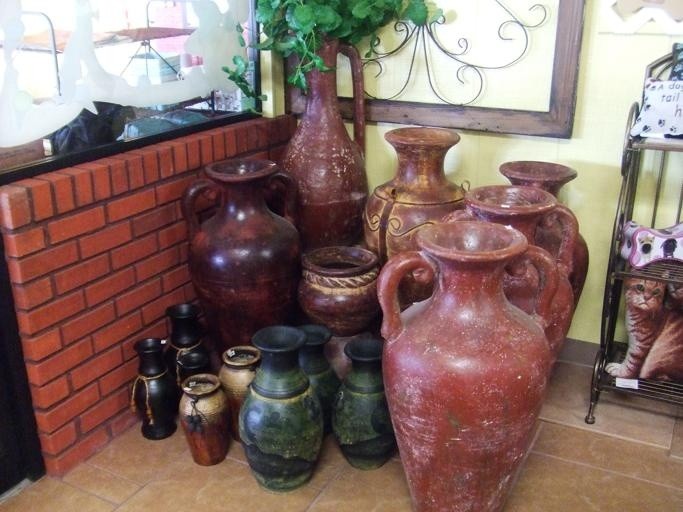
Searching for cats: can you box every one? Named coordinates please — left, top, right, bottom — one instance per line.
left=604, top=270, right=683, bottom=382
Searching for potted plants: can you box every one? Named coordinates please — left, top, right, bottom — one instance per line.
left=221, top=1, right=456, bottom=249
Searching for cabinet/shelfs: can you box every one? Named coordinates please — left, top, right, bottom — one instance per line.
left=586, top=43, right=683, bottom=424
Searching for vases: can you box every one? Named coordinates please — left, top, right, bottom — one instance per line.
left=180, top=156, right=307, bottom=346
left=219, top=346, right=261, bottom=443
left=131, top=338, right=178, bottom=441
left=179, top=373, right=231, bottom=465
left=378, top=217, right=556, bottom=512
left=301, top=321, right=334, bottom=429
left=302, top=244, right=382, bottom=381
left=362, top=130, right=469, bottom=264
left=336, top=334, right=399, bottom=469
left=463, top=182, right=583, bottom=368
left=498, top=159, right=589, bottom=307
left=237, top=319, right=327, bottom=493
left=167, top=302, right=220, bottom=381
